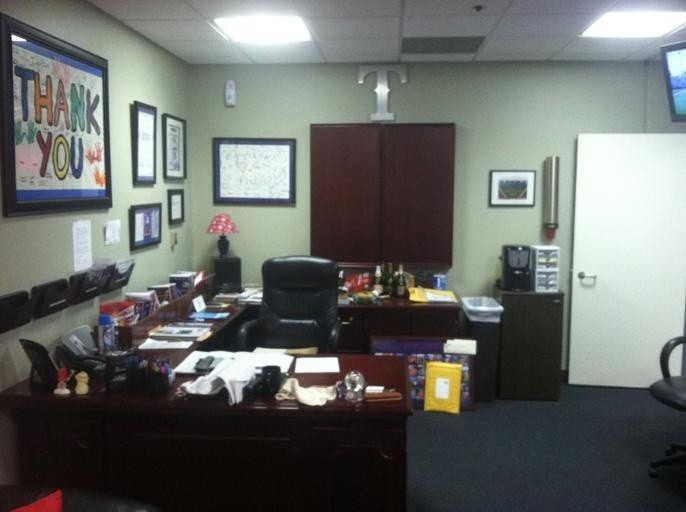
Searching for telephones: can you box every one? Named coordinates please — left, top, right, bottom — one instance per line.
left=71, top=355, right=110, bottom=375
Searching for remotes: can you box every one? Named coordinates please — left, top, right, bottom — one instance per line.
left=195, top=355, right=214, bottom=370
left=209, top=357, right=224, bottom=370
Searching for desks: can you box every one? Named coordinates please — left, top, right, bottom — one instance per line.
left=1, top=275, right=462, bottom=509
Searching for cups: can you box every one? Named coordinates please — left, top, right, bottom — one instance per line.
left=261, top=364, right=282, bottom=395
left=432, top=274, right=447, bottom=291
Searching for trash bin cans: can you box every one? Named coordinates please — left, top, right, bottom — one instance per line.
left=460, top=296, right=504, bottom=402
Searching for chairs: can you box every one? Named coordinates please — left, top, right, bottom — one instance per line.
left=647, top=335, right=686, bottom=477
left=236, top=254, right=344, bottom=354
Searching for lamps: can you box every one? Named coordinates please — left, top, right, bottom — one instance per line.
left=207, top=211, right=238, bottom=258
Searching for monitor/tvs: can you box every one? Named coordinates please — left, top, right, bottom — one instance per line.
left=660, top=41, right=686, bottom=122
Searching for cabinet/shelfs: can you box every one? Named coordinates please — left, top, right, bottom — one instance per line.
left=494, top=282, right=564, bottom=403
left=308, top=121, right=455, bottom=269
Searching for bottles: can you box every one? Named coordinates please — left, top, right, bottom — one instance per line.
left=374, top=261, right=406, bottom=298
left=337, top=268, right=346, bottom=288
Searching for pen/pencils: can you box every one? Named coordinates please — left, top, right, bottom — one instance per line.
left=148, top=358, right=171, bottom=375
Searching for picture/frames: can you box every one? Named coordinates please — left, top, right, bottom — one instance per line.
left=490, top=169, right=536, bottom=209
left=211, top=137, right=296, bottom=206
left=128, top=203, right=161, bottom=249
left=0, top=12, right=115, bottom=219
left=129, top=99, right=157, bottom=186
left=161, top=113, right=188, bottom=181
left=167, top=188, right=185, bottom=225
